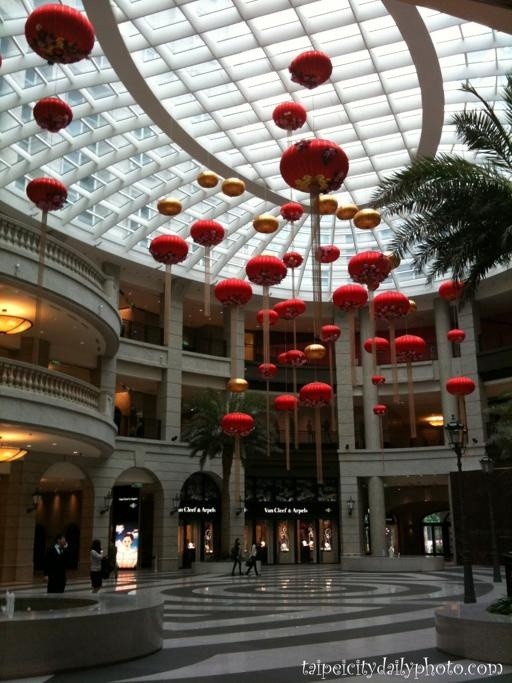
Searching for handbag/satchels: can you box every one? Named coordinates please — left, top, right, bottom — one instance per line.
left=245, top=560, right=253, bottom=568
left=99, top=556, right=114, bottom=579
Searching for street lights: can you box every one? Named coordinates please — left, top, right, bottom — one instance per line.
left=478, top=453, right=503, bottom=582
left=444, top=414, right=478, bottom=603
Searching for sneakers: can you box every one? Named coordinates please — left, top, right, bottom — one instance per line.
left=232, top=572, right=261, bottom=577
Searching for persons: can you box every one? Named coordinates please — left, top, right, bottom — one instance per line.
left=117, top=531, right=137, bottom=567
left=228, top=537, right=246, bottom=578
left=40, top=532, right=73, bottom=593
left=245, top=541, right=262, bottom=577
left=87, top=539, right=105, bottom=593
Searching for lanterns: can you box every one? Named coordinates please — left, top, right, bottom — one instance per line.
left=149, top=167, right=478, bottom=438
left=279, top=138, right=352, bottom=193
left=34, top=95, right=73, bottom=139
left=25, top=177, right=70, bottom=214
left=288, top=50, right=334, bottom=90
left=21, top=1, right=97, bottom=67
left=270, top=102, right=308, bottom=135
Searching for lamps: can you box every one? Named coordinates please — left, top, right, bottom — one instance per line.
left=28, top=487, right=40, bottom=513
left=429, top=420, right=443, bottom=427
left=0, top=314, right=34, bottom=335
left=0, top=446, right=28, bottom=462
left=346, top=496, right=355, bottom=516
left=236, top=496, right=245, bottom=514
left=170, top=492, right=180, bottom=515
left=100, top=490, right=113, bottom=515
